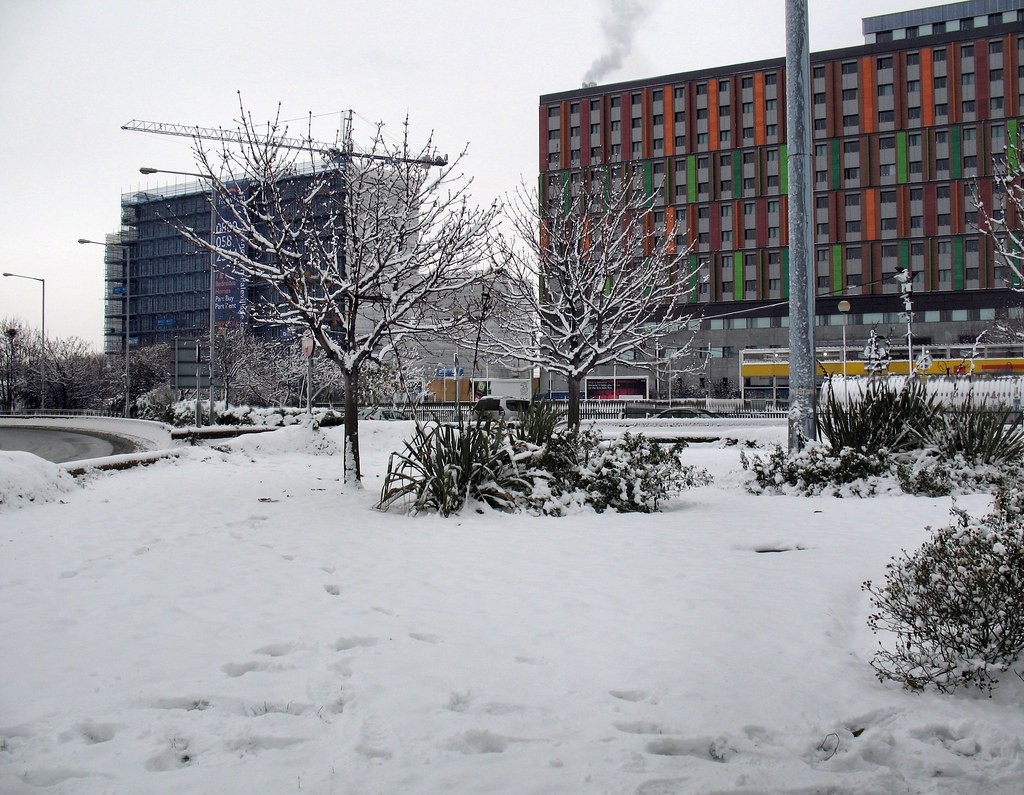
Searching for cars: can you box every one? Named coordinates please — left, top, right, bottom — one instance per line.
left=651, top=406, right=719, bottom=418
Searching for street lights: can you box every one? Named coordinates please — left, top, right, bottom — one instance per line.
left=78, top=239, right=129, bottom=417
left=140, top=166, right=217, bottom=425
left=3, top=273, right=45, bottom=408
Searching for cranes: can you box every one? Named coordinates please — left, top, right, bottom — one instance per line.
left=119, top=105, right=450, bottom=171
